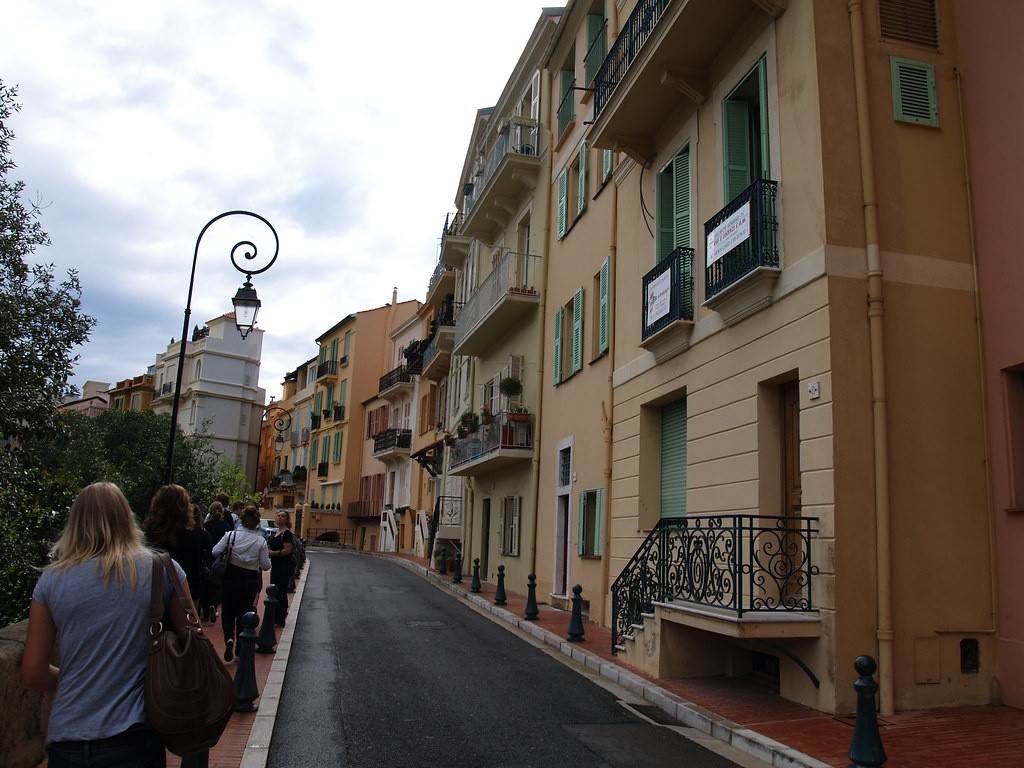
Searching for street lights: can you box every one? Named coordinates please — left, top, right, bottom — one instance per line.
left=164, top=208, right=280, bottom=485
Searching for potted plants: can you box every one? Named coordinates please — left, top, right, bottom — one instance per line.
left=436, top=556, right=451, bottom=570
left=500, top=377, right=522, bottom=396
left=507, top=406, right=532, bottom=422
left=458, top=413, right=479, bottom=439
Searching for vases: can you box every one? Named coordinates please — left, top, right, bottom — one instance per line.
left=481, top=415, right=494, bottom=424
left=444, top=435, right=455, bottom=446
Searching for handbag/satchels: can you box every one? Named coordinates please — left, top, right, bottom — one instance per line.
left=280, top=528, right=306, bottom=566
left=210, top=530, right=237, bottom=583
left=145, top=551, right=235, bottom=757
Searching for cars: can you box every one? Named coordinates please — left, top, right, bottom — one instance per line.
left=259, top=519, right=279, bottom=537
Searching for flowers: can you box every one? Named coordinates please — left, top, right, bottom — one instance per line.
left=481, top=406, right=489, bottom=415
left=438, top=428, right=449, bottom=435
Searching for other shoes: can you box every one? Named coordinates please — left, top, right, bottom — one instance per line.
left=200, top=615, right=211, bottom=622
left=223, top=638, right=234, bottom=663
left=235, top=705, right=258, bottom=713
left=209, top=605, right=217, bottom=623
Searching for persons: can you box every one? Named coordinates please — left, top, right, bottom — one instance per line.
left=22, top=481, right=206, bottom=768
left=212, top=506, right=271, bottom=664
left=268, top=509, right=293, bottom=627
left=192, top=492, right=245, bottom=623
left=142, top=483, right=200, bottom=612
